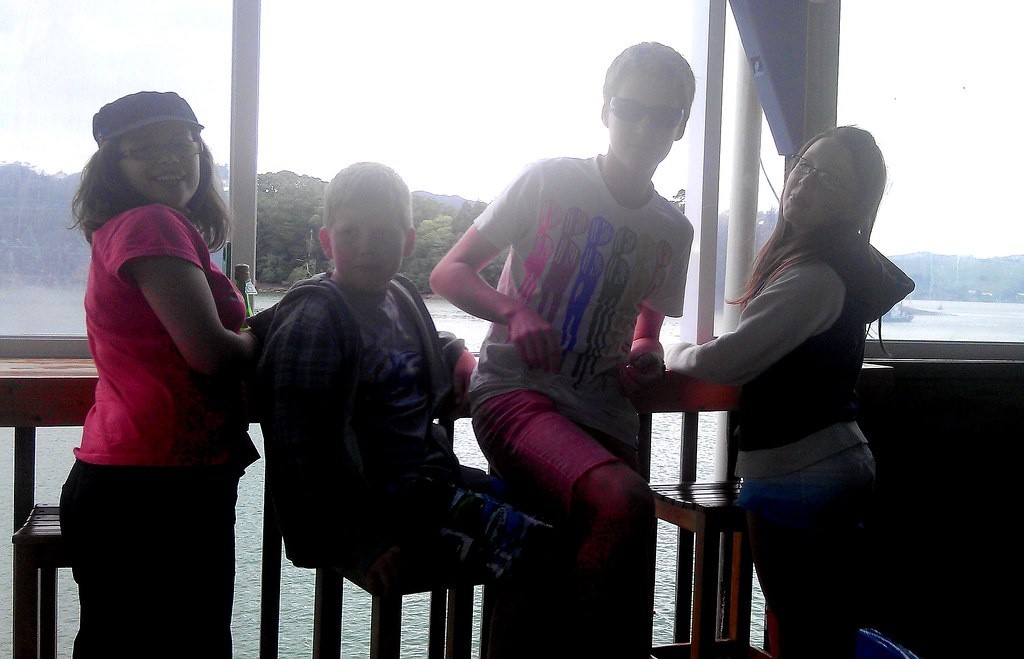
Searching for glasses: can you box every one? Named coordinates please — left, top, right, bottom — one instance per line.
left=119, top=141, right=204, bottom=160
left=609, top=96, right=686, bottom=130
left=789, top=154, right=861, bottom=199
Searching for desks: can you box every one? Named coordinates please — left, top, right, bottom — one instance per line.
left=0, top=359, right=893, bottom=659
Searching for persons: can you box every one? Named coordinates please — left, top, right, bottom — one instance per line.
left=663, top=124, right=916, bottom=659
left=57, top=90, right=258, bottom=658
left=431, top=42, right=694, bottom=659
left=244, top=162, right=562, bottom=659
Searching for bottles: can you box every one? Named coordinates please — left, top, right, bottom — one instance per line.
left=235, top=263, right=259, bottom=332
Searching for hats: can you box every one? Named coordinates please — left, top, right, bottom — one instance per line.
left=92, top=90, right=204, bottom=148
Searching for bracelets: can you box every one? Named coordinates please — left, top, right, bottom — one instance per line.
left=240, top=331, right=256, bottom=341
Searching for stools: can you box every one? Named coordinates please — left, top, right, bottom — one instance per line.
left=311, top=549, right=494, bottom=659
left=644, top=483, right=752, bottom=659
left=12, top=506, right=77, bottom=658
